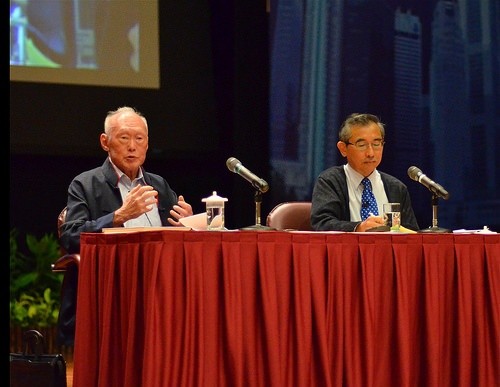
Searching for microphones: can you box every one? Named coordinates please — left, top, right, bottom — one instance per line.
left=407, top=165, right=449, bottom=200
left=226, top=157, right=269, bottom=194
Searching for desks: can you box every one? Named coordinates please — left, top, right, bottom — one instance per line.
left=71, top=230, right=500, bottom=387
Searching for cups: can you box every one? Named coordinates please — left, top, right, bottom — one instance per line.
left=205, top=201, right=224, bottom=231
left=383, top=203, right=401, bottom=231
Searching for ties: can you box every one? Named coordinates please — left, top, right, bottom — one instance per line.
left=361, top=177, right=379, bottom=222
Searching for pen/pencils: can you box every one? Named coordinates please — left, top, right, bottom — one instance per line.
left=368, top=211, right=375, bottom=216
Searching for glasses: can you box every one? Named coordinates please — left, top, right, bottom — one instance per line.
left=348, top=140, right=385, bottom=150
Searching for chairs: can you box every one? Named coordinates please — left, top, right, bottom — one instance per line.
left=50, top=206, right=80, bottom=274
left=266, top=202, right=312, bottom=230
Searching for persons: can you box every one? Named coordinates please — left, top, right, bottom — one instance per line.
left=309, top=112, right=419, bottom=234
left=56, top=106, right=193, bottom=363
